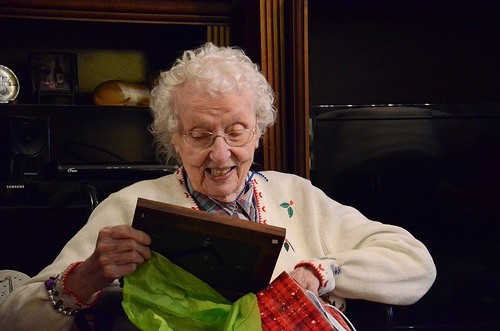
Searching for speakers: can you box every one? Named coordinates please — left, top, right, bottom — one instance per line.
left=0, top=114, right=51, bottom=184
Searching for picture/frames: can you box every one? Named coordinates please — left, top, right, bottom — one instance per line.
left=118, top=198, right=285, bottom=303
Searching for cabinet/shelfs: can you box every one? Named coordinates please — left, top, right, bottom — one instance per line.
left=0, top=0, right=234, bottom=331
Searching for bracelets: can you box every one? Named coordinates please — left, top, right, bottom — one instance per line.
left=45, top=274, right=80, bottom=316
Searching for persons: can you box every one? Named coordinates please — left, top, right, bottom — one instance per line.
left=0, top=41, right=438, bottom=331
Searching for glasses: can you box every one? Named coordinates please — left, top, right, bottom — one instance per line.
left=181, top=129, right=258, bottom=148
